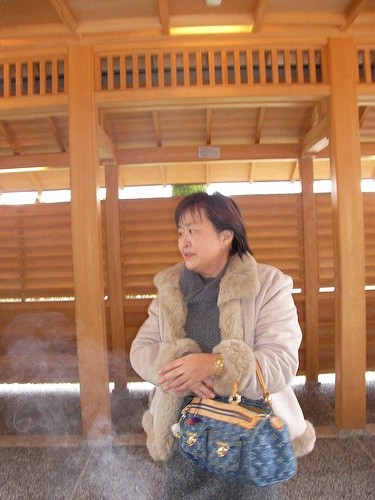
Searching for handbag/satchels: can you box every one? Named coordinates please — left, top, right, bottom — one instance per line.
left=171, top=356, right=297, bottom=486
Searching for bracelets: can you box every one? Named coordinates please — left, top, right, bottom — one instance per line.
left=215, top=353, right=225, bottom=377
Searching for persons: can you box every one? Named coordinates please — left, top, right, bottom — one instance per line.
left=129, top=192, right=316, bottom=500
